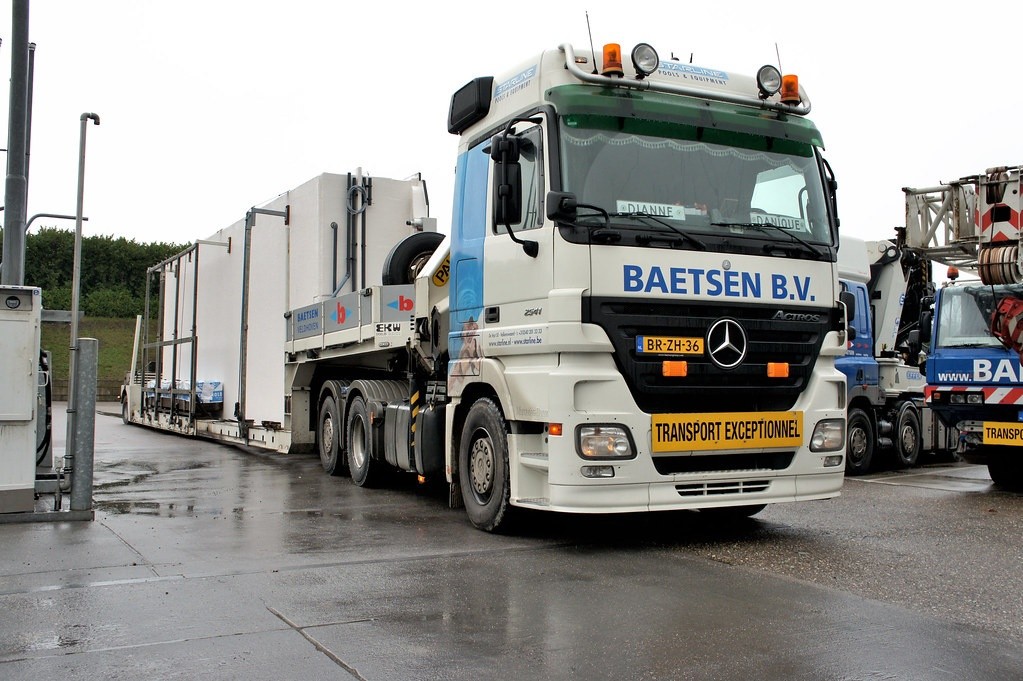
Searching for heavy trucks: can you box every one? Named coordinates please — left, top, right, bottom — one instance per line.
left=839, top=165, right=1022, bottom=493
left=119, top=11, right=849, bottom=532
